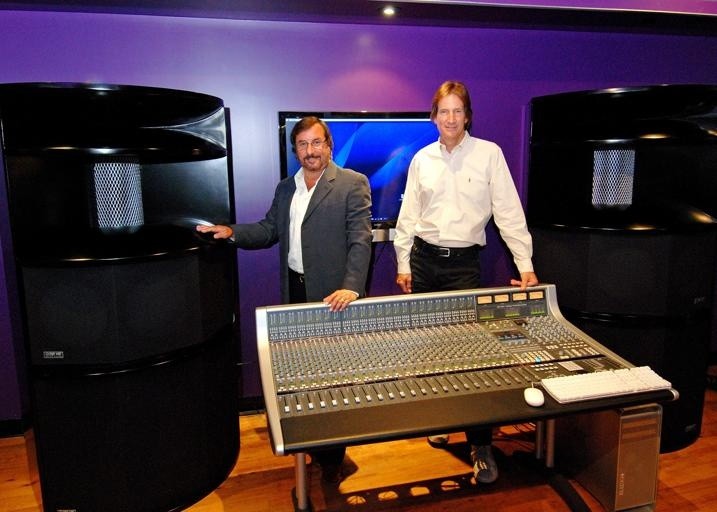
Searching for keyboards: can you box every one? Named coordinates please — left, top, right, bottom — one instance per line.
left=540, top=365, right=673, bottom=404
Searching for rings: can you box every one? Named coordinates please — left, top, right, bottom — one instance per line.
left=340, top=298, right=344, bottom=302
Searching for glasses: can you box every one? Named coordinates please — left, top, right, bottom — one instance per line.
left=298, top=141, right=323, bottom=148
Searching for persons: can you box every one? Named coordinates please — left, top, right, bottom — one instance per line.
left=392, top=81, right=539, bottom=483
left=195, top=118, right=371, bottom=484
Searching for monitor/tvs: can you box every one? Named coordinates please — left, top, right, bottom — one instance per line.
left=276, top=110, right=471, bottom=243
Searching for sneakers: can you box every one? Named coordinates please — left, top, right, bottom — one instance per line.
left=428, top=433, right=451, bottom=446
left=471, top=442, right=497, bottom=482
left=309, top=449, right=342, bottom=485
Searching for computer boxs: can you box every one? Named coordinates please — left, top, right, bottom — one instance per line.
left=555, top=401, right=666, bottom=511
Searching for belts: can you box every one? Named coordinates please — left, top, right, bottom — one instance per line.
left=414, top=237, right=477, bottom=257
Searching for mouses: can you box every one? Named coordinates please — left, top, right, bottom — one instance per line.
left=524, top=387, right=545, bottom=407
